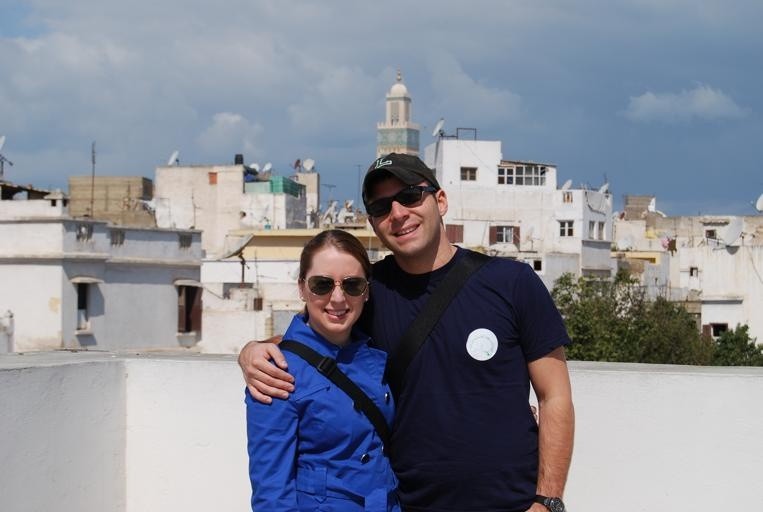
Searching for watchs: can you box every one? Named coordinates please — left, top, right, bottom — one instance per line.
left=535, top=495, right=565, bottom=512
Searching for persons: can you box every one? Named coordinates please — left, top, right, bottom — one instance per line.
left=244, top=229, right=539, bottom=512
left=237, top=153, right=575, bottom=512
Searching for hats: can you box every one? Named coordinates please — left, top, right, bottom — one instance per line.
left=362, top=153, right=440, bottom=203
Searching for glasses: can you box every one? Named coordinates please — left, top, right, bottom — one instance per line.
left=364, top=186, right=436, bottom=217
left=303, top=276, right=370, bottom=296
